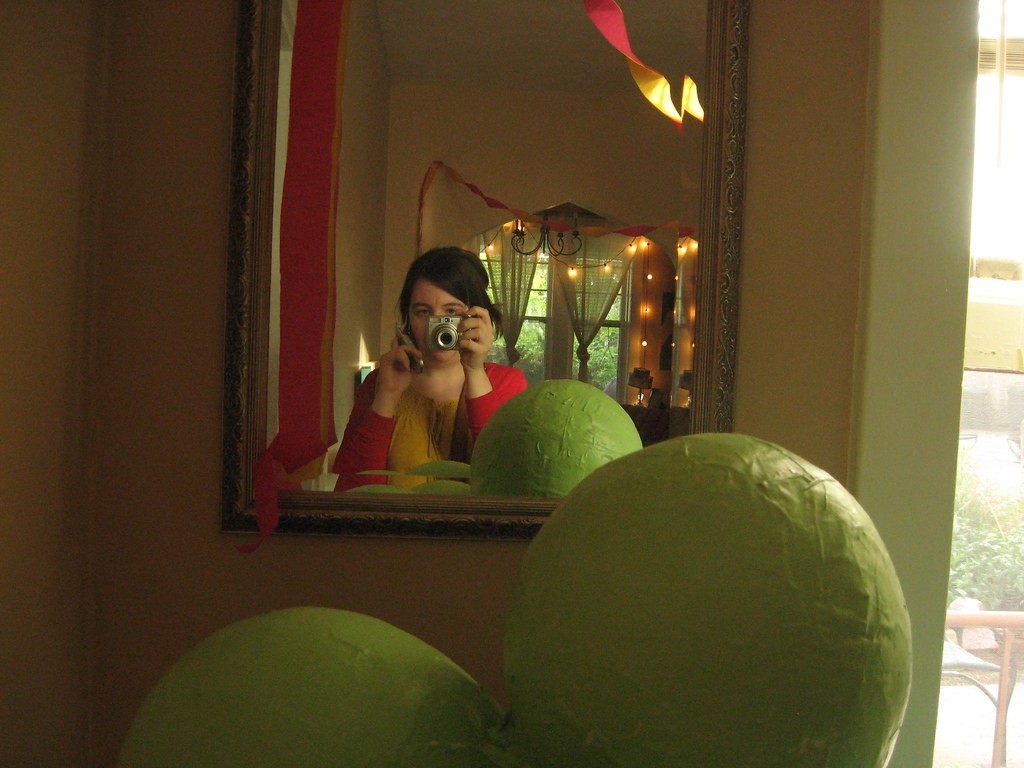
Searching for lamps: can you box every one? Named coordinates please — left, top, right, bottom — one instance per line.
left=511, top=207, right=582, bottom=257
left=627, top=367, right=653, bottom=407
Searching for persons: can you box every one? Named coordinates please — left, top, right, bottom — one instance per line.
left=333, top=247, right=529, bottom=498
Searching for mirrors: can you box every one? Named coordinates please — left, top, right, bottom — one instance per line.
left=218, top=0, right=753, bottom=542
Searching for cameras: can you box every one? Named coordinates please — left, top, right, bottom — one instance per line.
left=427, top=315, right=474, bottom=351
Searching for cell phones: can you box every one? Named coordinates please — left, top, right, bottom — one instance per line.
left=398, top=326, right=424, bottom=374
